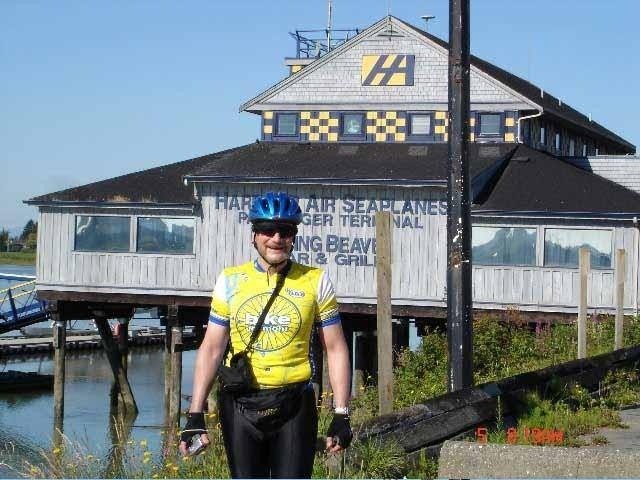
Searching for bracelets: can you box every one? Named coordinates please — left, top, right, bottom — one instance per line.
left=333, top=408, right=350, bottom=414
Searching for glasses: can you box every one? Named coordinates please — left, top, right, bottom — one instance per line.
left=251, top=227, right=299, bottom=239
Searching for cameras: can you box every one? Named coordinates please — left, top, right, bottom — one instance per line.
left=186, top=434, right=211, bottom=457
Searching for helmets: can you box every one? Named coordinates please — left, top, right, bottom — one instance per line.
left=247, top=193, right=302, bottom=225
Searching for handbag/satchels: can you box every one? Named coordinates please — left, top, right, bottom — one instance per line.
left=236, top=385, right=301, bottom=430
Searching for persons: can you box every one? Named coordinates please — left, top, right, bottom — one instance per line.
left=178, top=190, right=353, bottom=479
